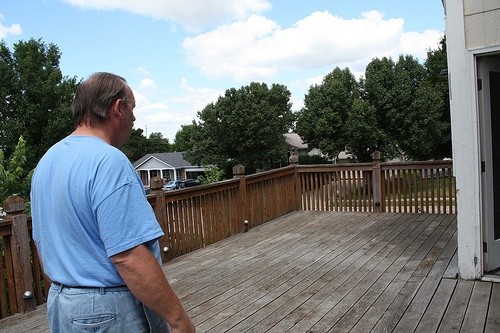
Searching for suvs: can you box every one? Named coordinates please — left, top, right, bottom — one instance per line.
left=175, top=178, right=200, bottom=190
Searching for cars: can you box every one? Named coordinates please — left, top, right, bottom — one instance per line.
left=162, top=179, right=181, bottom=191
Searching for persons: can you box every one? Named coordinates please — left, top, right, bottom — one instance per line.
left=29, top=71, right=196, bottom=333
